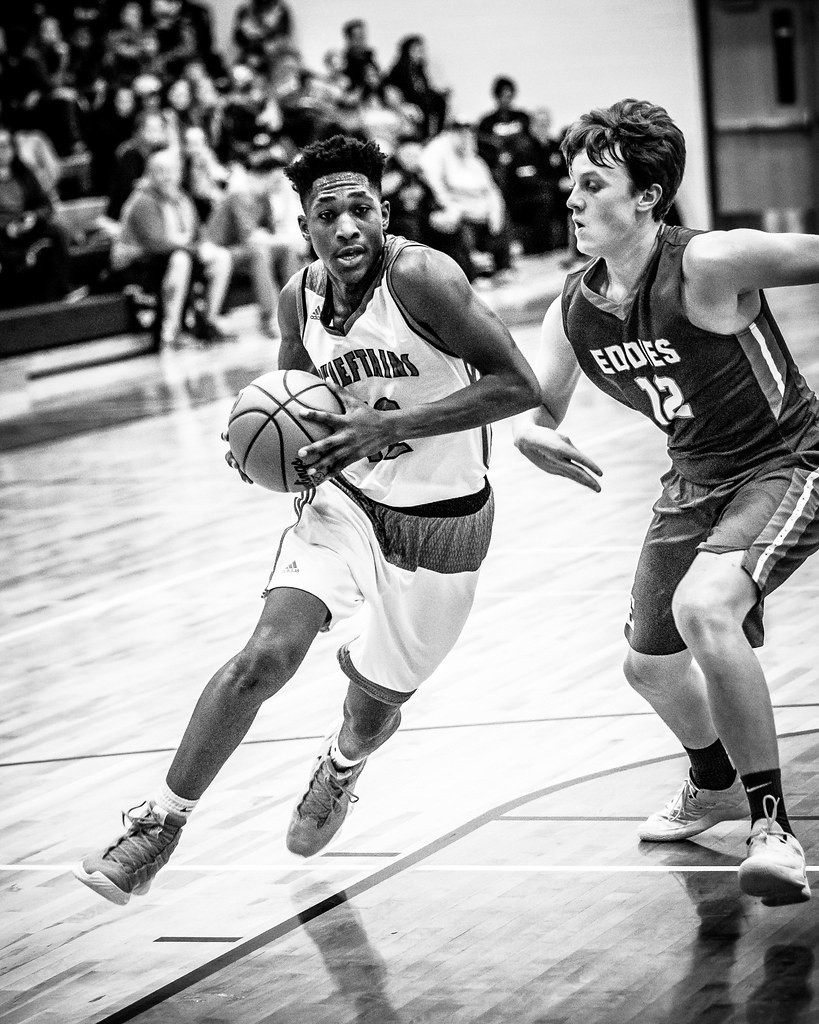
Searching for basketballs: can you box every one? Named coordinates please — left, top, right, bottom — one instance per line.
left=226, top=368, right=344, bottom=494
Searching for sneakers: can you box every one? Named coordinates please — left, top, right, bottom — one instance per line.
left=286, top=726, right=370, bottom=857
left=738, top=792, right=810, bottom=908
left=636, top=765, right=750, bottom=840
left=70, top=800, right=187, bottom=907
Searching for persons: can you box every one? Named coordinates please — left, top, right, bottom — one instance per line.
left=512, top=97, right=817, bottom=908
left=0, top=0, right=576, bottom=352
left=634, top=840, right=819, bottom=1024
left=74, top=133, right=545, bottom=907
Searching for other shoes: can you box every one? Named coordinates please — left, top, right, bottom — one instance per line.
left=192, top=318, right=239, bottom=344
left=256, top=317, right=279, bottom=339
left=488, top=267, right=520, bottom=283
left=472, top=277, right=494, bottom=291
left=157, top=337, right=203, bottom=355
